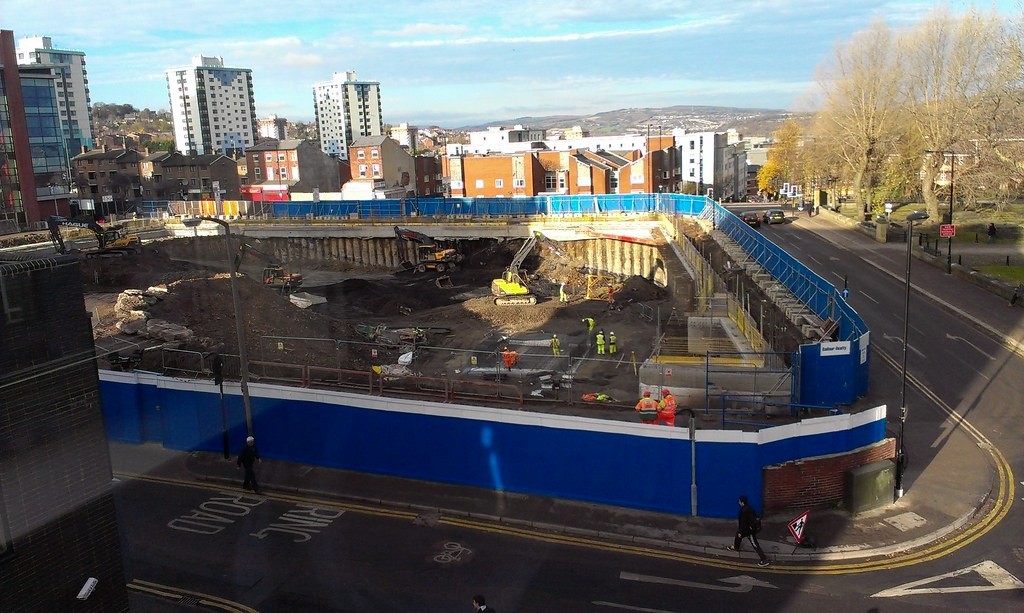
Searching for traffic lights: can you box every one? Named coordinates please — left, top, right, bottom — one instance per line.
left=707, top=188, right=713, bottom=199
left=791, top=186, right=797, bottom=197
left=784, top=183, right=789, bottom=194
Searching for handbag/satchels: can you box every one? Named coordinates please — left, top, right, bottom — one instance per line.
left=754, top=517, right=762, bottom=533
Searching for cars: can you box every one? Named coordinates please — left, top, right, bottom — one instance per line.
left=763, top=209, right=786, bottom=225
left=737, top=211, right=761, bottom=228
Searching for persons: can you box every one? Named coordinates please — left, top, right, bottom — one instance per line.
left=1008, top=288, right=1019, bottom=307
left=635, top=391, right=661, bottom=425
left=237, top=436, right=262, bottom=496
left=596, top=331, right=605, bottom=355
left=608, top=283, right=617, bottom=305
left=560, top=282, right=569, bottom=302
left=550, top=335, right=560, bottom=358
left=506, top=268, right=513, bottom=283
left=501, top=347, right=519, bottom=372
left=807, top=201, right=813, bottom=217
left=658, top=389, right=677, bottom=426
left=609, top=332, right=617, bottom=354
left=727, top=496, right=769, bottom=566
left=582, top=318, right=595, bottom=335
left=988, top=223, right=997, bottom=243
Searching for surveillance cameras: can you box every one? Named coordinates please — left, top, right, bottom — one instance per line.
left=76, top=577, right=98, bottom=600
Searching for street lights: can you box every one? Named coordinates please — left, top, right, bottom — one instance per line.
left=923, top=150, right=954, bottom=274
left=659, top=126, right=661, bottom=182
left=648, top=124, right=653, bottom=210
left=182, top=217, right=253, bottom=437
left=48, top=182, right=59, bottom=217
left=895, top=213, right=930, bottom=497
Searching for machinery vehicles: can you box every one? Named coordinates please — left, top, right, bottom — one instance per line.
left=491, top=231, right=570, bottom=306
left=233, top=242, right=303, bottom=296
left=394, top=226, right=462, bottom=273
left=47, top=215, right=142, bottom=259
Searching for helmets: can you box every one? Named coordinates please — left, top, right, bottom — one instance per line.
left=582, top=319, right=585, bottom=322
left=553, top=334, right=557, bottom=338
left=599, top=331, right=603, bottom=333
left=610, top=332, right=614, bottom=336
left=661, top=390, right=669, bottom=396
left=247, top=436, right=254, bottom=442
left=643, top=391, right=650, bottom=396
left=504, top=347, right=507, bottom=349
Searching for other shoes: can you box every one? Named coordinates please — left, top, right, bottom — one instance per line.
left=255, top=489, right=264, bottom=494
left=757, top=560, right=769, bottom=567
left=726, top=545, right=739, bottom=553
